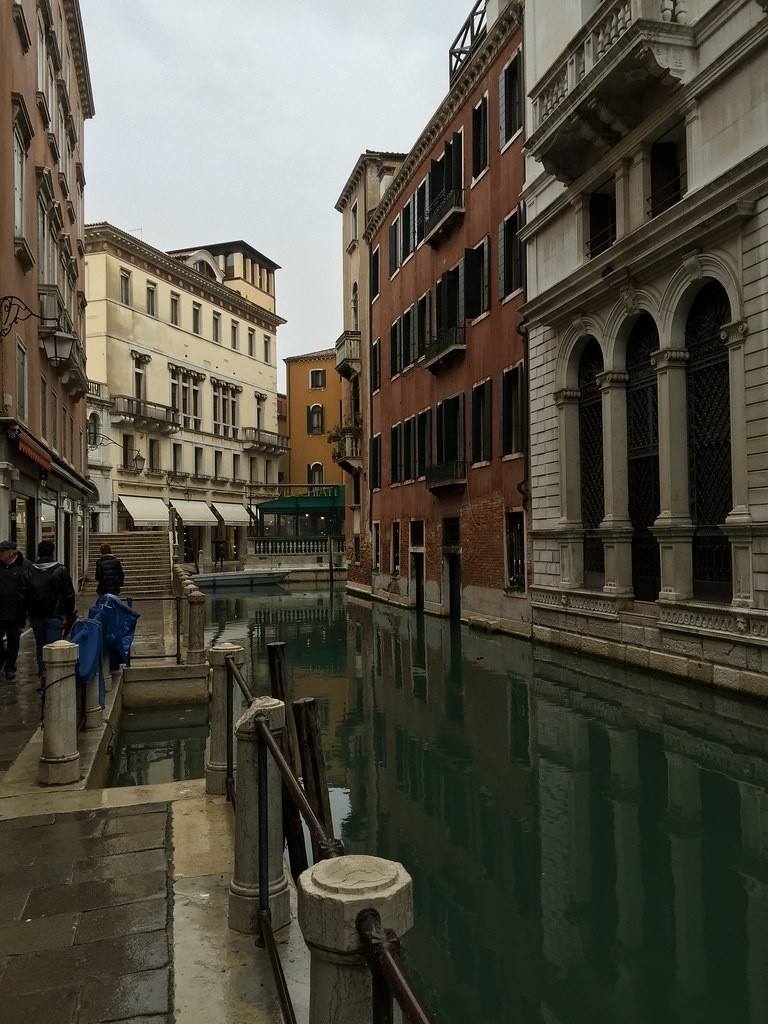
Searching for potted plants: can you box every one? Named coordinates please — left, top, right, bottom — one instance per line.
left=338, top=442, right=346, bottom=457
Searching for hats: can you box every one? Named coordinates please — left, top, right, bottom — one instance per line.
left=0, top=540, right=17, bottom=551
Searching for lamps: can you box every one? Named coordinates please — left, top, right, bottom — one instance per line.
left=0, top=296, right=77, bottom=372
left=86, top=431, right=146, bottom=474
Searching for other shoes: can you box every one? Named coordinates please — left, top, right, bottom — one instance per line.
left=0, top=647, right=7, bottom=671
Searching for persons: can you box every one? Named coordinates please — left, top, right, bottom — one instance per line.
left=19, top=541, right=76, bottom=687
left=214, top=542, right=224, bottom=567
left=95, top=543, right=125, bottom=598
left=0, top=541, right=32, bottom=679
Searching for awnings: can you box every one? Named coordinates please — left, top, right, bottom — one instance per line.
left=118, top=496, right=285, bottom=527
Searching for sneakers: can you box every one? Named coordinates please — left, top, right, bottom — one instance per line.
left=39, top=671, right=47, bottom=690
left=5, top=671, right=17, bottom=680
